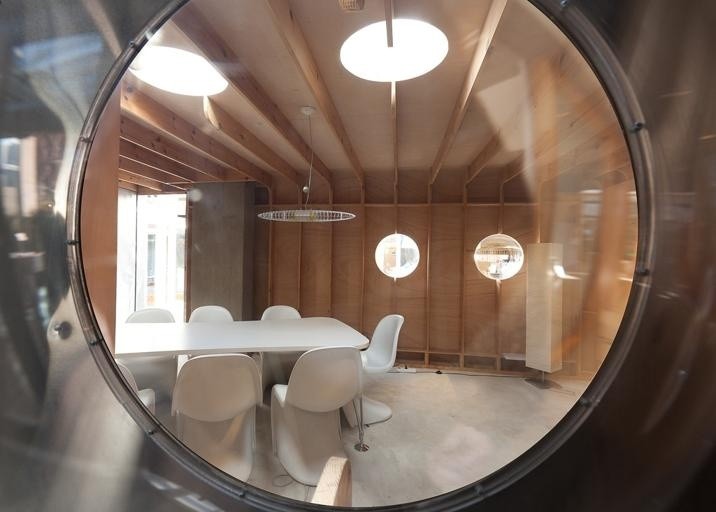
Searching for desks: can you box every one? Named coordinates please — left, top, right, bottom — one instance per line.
left=115, top=316, right=372, bottom=454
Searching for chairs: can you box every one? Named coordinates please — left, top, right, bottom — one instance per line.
left=115, top=363, right=155, bottom=418
left=271, top=344, right=365, bottom=489
left=169, top=352, right=263, bottom=484
left=186, top=305, right=236, bottom=360
left=125, top=308, right=178, bottom=440
left=340, top=314, right=406, bottom=428
left=258, top=305, right=302, bottom=408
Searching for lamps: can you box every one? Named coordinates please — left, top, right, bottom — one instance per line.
left=472, top=230, right=527, bottom=287
left=253, top=104, right=360, bottom=225
left=374, top=231, right=421, bottom=284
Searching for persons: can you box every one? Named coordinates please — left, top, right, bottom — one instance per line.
left=30, top=191, right=69, bottom=314
